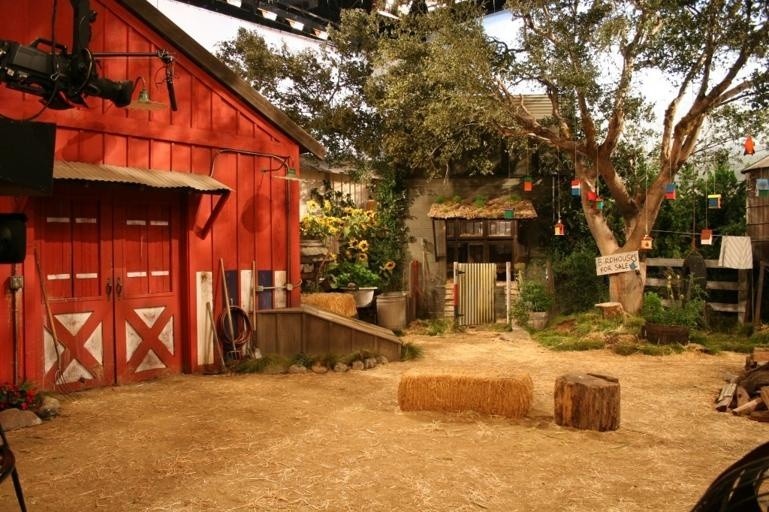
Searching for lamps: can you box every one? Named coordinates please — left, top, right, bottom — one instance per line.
left=260, top=153, right=305, bottom=184
left=126, top=74, right=167, bottom=113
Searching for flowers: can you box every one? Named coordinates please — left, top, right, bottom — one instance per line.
left=300, top=194, right=398, bottom=276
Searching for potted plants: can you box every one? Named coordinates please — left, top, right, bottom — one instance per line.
left=642, top=287, right=705, bottom=349
left=332, top=260, right=386, bottom=311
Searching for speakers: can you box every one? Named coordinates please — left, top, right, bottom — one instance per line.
left=0, top=212, right=27, bottom=264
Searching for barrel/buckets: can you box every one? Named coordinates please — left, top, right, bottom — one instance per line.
left=645, top=321, right=688, bottom=346
left=529, top=311, right=550, bottom=329
